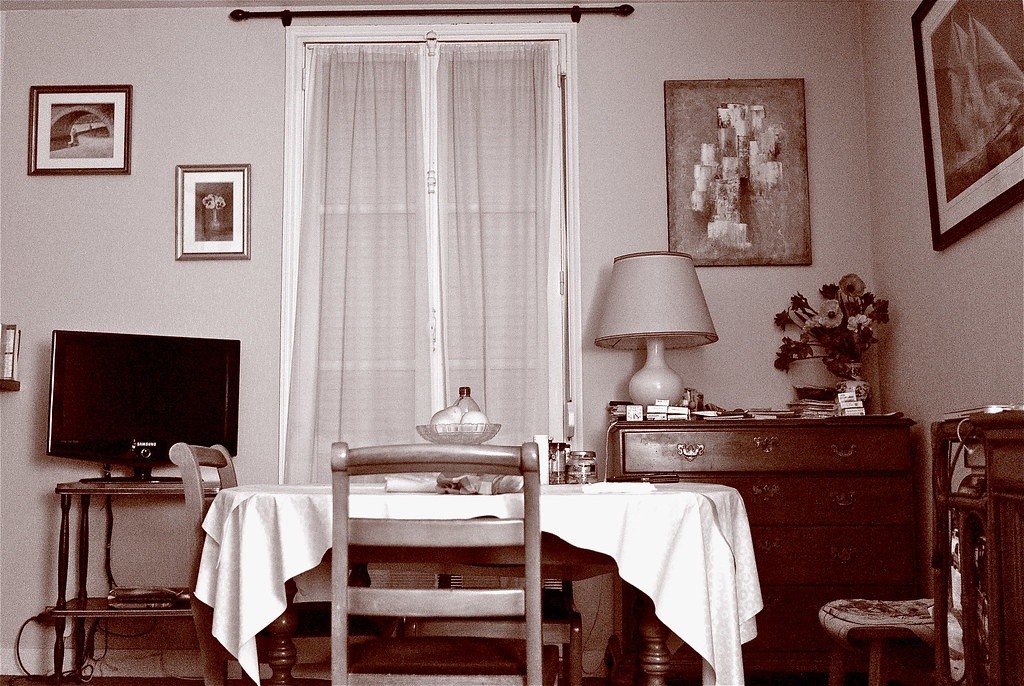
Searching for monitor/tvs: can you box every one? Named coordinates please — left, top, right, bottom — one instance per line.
left=46, top=329, right=241, bottom=484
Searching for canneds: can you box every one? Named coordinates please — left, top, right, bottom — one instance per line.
left=549, top=442, right=598, bottom=486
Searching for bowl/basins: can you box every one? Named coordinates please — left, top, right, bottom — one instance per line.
left=416, top=423, right=501, bottom=445
left=792, top=384, right=838, bottom=401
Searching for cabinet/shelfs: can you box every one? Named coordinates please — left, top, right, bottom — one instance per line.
left=931, top=411, right=1024, bottom=686
left=608, top=418, right=930, bottom=685
left=48, top=481, right=223, bottom=684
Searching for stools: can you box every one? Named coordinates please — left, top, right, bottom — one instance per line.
left=817, top=599, right=933, bottom=684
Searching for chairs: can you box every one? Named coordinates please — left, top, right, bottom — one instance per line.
left=167, top=443, right=583, bottom=686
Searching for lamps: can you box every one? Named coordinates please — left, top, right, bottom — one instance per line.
left=594, top=255, right=720, bottom=408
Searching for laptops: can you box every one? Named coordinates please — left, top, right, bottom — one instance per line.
left=944, top=404, right=1024, bottom=421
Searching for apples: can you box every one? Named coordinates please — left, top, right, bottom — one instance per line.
left=461, top=412, right=488, bottom=432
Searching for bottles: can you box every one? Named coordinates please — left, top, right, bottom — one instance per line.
left=566, top=450, right=598, bottom=484
left=549, top=442, right=566, bottom=485
left=452, top=386, right=482, bottom=417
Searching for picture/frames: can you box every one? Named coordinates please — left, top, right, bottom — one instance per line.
left=174, top=164, right=253, bottom=262
left=28, top=85, right=133, bottom=177
left=911, top=0, right=1024, bottom=252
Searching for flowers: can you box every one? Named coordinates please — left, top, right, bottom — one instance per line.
left=774, top=273, right=890, bottom=381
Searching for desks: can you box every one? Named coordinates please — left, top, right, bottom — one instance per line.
left=193, top=482, right=763, bottom=686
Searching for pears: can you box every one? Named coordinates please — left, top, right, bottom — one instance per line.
left=430, top=406, right=460, bottom=431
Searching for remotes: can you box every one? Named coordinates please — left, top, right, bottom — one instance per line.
left=609, top=473, right=678, bottom=482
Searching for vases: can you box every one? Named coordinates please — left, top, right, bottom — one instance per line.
left=835, top=362, right=871, bottom=404
